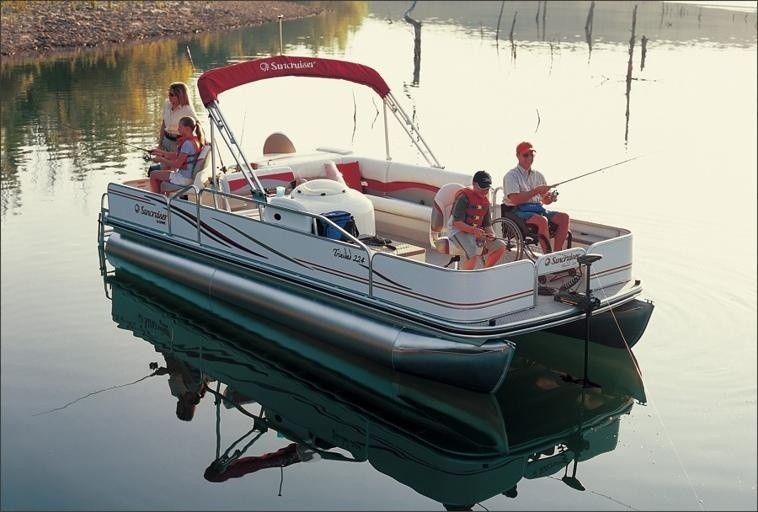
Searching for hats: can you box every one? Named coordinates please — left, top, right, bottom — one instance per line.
left=473, top=170, right=492, bottom=188
left=516, top=141, right=537, bottom=153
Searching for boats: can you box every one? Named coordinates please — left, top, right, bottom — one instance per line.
left=100, top=55, right=654, bottom=393
left=96, top=250, right=650, bottom=512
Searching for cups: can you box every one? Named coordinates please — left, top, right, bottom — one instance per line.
left=277, top=186, right=286, bottom=197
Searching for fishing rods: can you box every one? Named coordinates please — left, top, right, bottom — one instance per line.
left=62, top=123, right=152, bottom=153
left=485, top=234, right=595, bottom=266
left=548, top=157, right=639, bottom=188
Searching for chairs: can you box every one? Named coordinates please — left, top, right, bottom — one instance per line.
left=161, top=144, right=212, bottom=199
left=431, top=183, right=492, bottom=270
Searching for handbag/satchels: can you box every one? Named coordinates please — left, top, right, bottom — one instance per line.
left=311, top=211, right=359, bottom=244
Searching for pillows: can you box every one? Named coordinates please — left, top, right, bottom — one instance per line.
left=327, top=161, right=363, bottom=193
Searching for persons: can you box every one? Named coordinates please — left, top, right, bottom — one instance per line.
left=503, top=141, right=570, bottom=254
left=149, top=115, right=204, bottom=195
left=163, top=351, right=212, bottom=421
left=155, top=82, right=209, bottom=197
left=446, top=170, right=508, bottom=271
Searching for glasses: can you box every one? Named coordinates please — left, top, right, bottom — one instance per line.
left=522, top=154, right=534, bottom=157
left=168, top=92, right=178, bottom=97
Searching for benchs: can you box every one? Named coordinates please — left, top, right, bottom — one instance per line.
left=289, top=156, right=474, bottom=223
